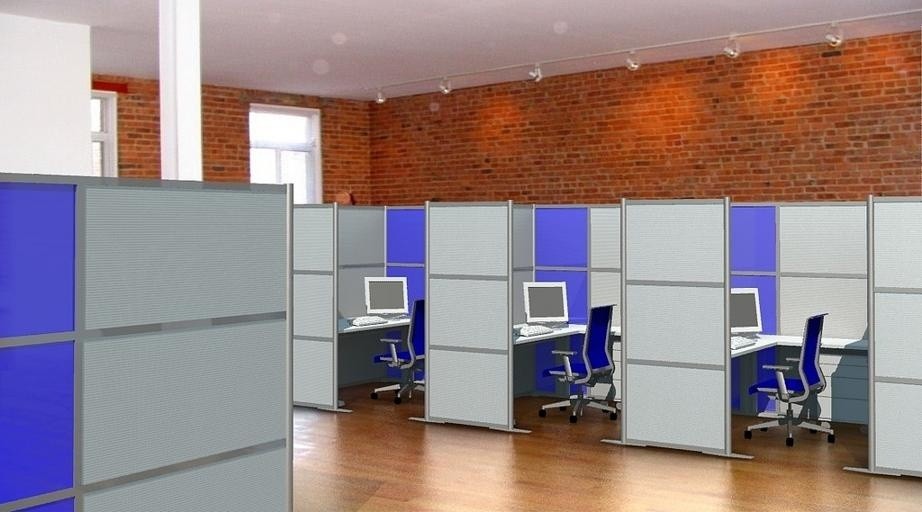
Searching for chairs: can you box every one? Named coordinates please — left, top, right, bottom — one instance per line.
left=371, top=299, right=426, bottom=405
left=538, top=303, right=620, bottom=418
left=742, top=313, right=835, bottom=446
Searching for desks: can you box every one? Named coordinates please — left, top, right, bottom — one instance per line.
left=513, top=324, right=622, bottom=345
left=337, top=315, right=411, bottom=334
left=731, top=334, right=868, bottom=359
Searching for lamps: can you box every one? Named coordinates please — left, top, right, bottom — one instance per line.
left=375, top=16, right=843, bottom=105
